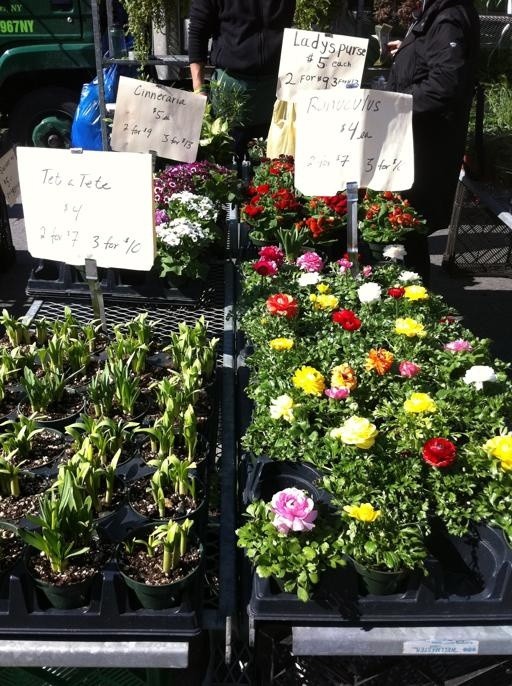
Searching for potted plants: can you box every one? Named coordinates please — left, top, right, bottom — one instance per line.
left=1, top=308, right=222, bottom=617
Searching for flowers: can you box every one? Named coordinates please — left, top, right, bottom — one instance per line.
left=234, top=138, right=426, bottom=255
left=150, top=155, right=240, bottom=284
left=238, top=247, right=511, bottom=602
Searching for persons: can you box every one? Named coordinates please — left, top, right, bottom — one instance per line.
left=371, top=1, right=481, bottom=295
left=189, top=0, right=297, bottom=175
